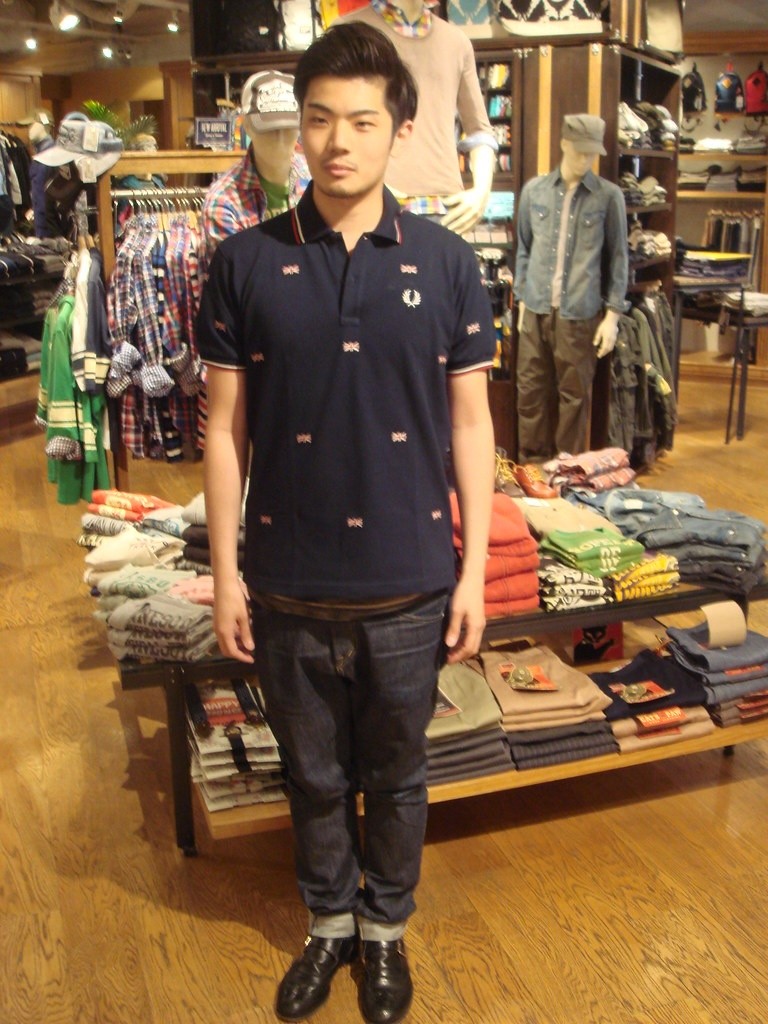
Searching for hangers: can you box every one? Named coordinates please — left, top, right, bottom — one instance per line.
left=65, top=186, right=205, bottom=251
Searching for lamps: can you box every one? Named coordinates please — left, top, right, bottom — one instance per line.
left=168, top=10, right=181, bottom=31
left=48, top=0, right=80, bottom=31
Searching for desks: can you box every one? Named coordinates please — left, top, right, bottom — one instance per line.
left=681, top=305, right=768, bottom=441
left=673, top=275, right=748, bottom=444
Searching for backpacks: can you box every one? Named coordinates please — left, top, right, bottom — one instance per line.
left=743, top=64, right=768, bottom=138
left=714, top=63, right=746, bottom=133
left=681, top=66, right=707, bottom=132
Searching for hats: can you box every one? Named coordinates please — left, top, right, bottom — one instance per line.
left=241, top=69, right=301, bottom=131
left=33, top=120, right=124, bottom=167
left=561, top=114, right=606, bottom=156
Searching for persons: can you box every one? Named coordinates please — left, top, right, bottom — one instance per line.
left=118, top=134, right=166, bottom=206
left=512, top=113, right=629, bottom=466
left=29, top=123, right=62, bottom=239
left=198, top=69, right=301, bottom=307
left=192, top=20, right=497, bottom=1024
left=329, top=1, right=499, bottom=235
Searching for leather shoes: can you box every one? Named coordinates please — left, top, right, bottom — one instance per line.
left=276, top=926, right=412, bottom=1024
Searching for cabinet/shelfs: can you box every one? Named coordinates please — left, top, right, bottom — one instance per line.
left=117, top=571, right=768, bottom=857
left=161, top=33, right=768, bottom=464
